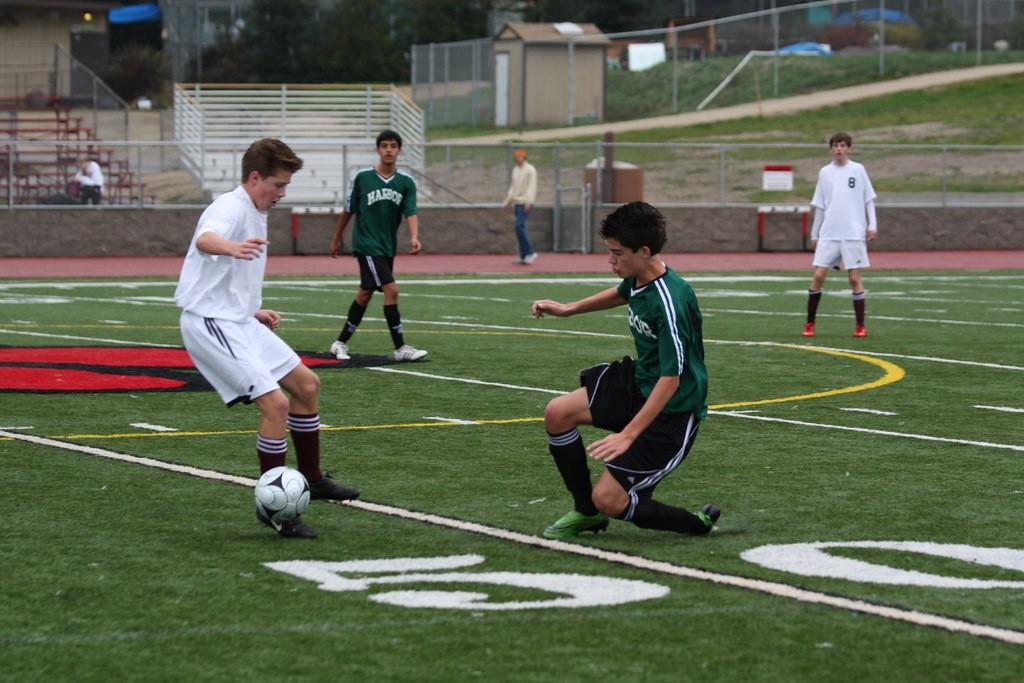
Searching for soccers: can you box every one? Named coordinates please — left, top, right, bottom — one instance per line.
left=254, top=465, right=312, bottom=524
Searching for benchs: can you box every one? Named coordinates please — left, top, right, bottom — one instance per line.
left=0, top=95, right=146, bottom=206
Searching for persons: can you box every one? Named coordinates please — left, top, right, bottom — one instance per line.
left=532, top=201, right=721, bottom=539
left=503, top=149, right=537, bottom=265
left=174, top=139, right=359, bottom=538
left=67, top=152, right=105, bottom=205
left=801, top=133, right=878, bottom=338
left=329, top=130, right=428, bottom=363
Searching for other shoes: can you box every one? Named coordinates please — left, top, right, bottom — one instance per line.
left=853, top=325, right=866, bottom=336
left=803, top=323, right=814, bottom=336
left=512, top=258, right=523, bottom=263
left=523, top=252, right=537, bottom=263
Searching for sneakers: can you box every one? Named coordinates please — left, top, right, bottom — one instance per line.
left=394, top=346, right=428, bottom=361
left=256, top=508, right=317, bottom=538
left=329, top=341, right=351, bottom=360
left=542, top=510, right=608, bottom=538
left=692, top=504, right=721, bottom=530
left=309, top=477, right=358, bottom=499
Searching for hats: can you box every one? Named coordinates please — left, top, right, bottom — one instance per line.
left=515, top=149, right=525, bottom=157
